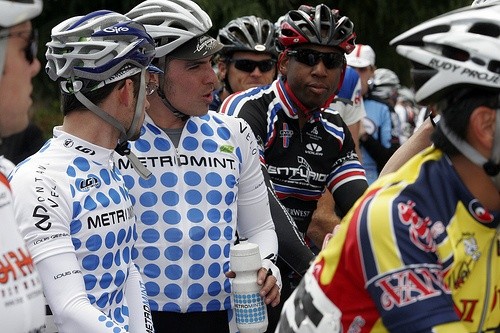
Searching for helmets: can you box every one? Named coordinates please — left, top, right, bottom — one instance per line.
left=123, top=0, right=212, bottom=59
left=216, top=15, right=279, bottom=64
left=368, top=68, right=399, bottom=97
left=45, top=10, right=156, bottom=82
left=395, top=85, right=417, bottom=106
left=390, top=0, right=500, bottom=107
left=274, top=4, right=356, bottom=65
left=0, top=0, right=43, bottom=28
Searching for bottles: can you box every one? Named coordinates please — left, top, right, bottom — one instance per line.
left=229, top=237, right=268, bottom=333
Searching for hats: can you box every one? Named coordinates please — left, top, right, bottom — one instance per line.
left=127, top=64, right=164, bottom=79
left=152, top=32, right=224, bottom=61
left=343, top=44, right=374, bottom=68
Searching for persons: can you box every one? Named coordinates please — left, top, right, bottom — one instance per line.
left=7, top=9, right=166, bottom=333
left=219, top=3, right=368, bottom=333
left=114, top=0, right=283, bottom=333
left=0, top=0, right=500, bottom=333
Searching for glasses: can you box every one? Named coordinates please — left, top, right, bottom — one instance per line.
left=285, top=48, right=345, bottom=69
left=0, top=30, right=38, bottom=66
left=116, top=73, right=159, bottom=96
left=349, top=65, right=371, bottom=72
left=228, top=58, right=278, bottom=73
